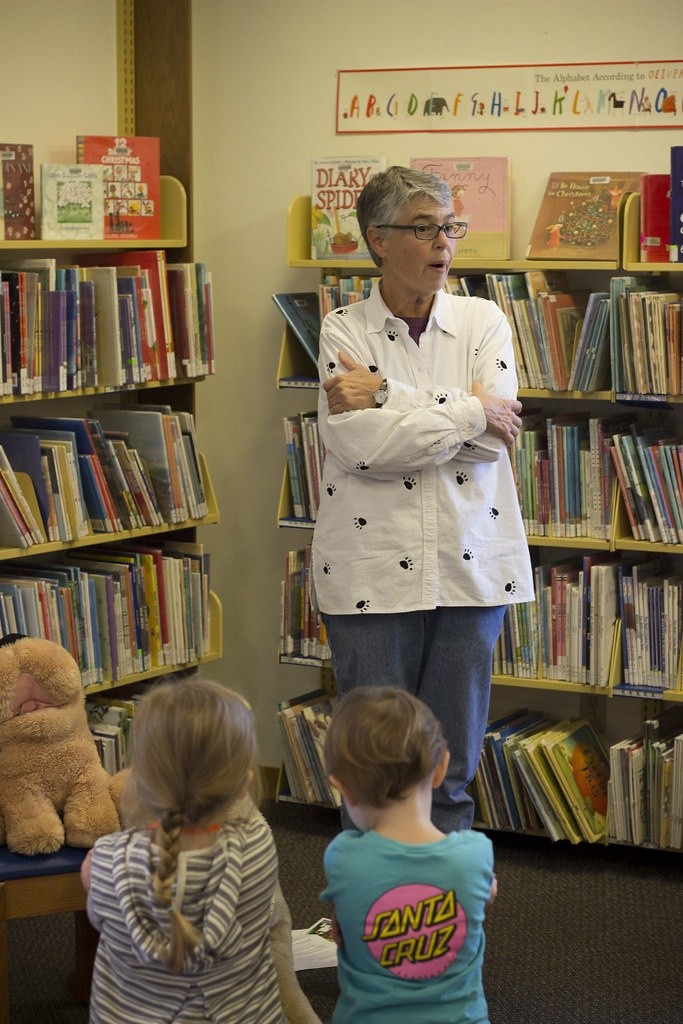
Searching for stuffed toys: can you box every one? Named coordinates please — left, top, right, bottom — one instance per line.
left=0, top=638, right=132, bottom=854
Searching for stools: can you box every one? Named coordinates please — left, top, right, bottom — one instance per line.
left=0, top=834, right=101, bottom=1024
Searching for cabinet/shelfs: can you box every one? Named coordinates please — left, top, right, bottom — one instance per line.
left=0, top=175, right=223, bottom=695
left=608, top=196, right=683, bottom=858
left=274, top=194, right=608, bottom=849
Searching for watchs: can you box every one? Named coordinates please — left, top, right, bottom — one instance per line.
left=373, top=378, right=388, bottom=407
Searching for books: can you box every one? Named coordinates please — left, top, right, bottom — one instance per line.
left=276, top=689, right=344, bottom=808
left=0, top=403, right=208, bottom=548
left=463, top=707, right=683, bottom=850
left=283, top=412, right=326, bottom=522
left=0, top=250, right=214, bottom=396
left=491, top=412, right=683, bottom=694
left=85, top=693, right=145, bottom=777
left=0, top=135, right=161, bottom=241
left=282, top=543, right=332, bottom=661
left=270, top=275, right=383, bottom=370
left=439, top=273, right=683, bottom=396
left=310, top=146, right=683, bottom=263
left=0, top=542, right=211, bottom=688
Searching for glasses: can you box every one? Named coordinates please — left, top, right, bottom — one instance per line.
left=374, top=221, right=467, bottom=240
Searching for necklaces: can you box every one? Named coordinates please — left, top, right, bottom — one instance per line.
left=149, top=822, right=220, bottom=833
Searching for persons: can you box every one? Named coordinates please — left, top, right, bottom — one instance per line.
left=310, top=165, right=536, bottom=836
left=79, top=678, right=290, bottom=1024
left=316, top=687, right=498, bottom=1024
